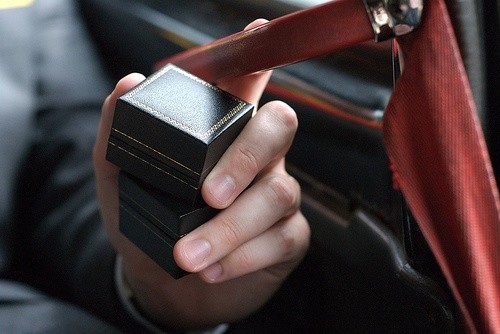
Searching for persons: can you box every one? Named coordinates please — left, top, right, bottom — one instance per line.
left=0, top=0, right=312, bottom=334
